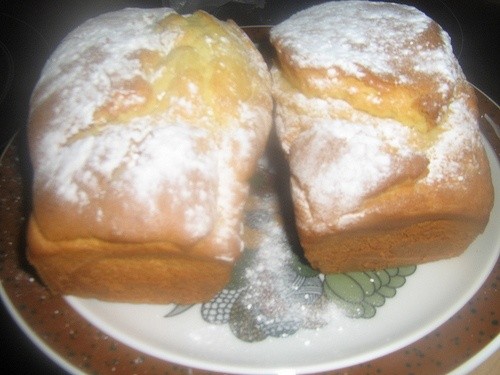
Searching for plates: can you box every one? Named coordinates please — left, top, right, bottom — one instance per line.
left=0, top=24, right=500, bottom=375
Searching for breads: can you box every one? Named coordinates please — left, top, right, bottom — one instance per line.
left=264, top=0, right=494, bottom=273
left=25, top=8, right=274, bottom=306
